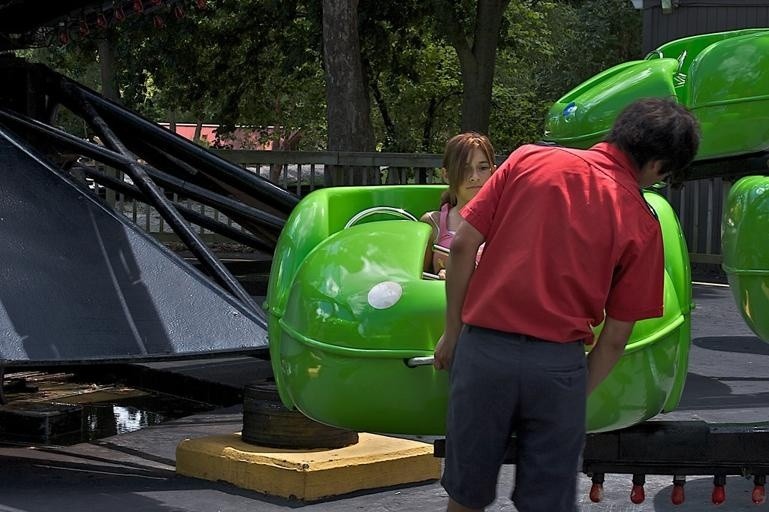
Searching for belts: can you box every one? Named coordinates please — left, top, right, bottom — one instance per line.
left=462, top=321, right=552, bottom=346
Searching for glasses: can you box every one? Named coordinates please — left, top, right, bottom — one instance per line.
left=651, top=177, right=673, bottom=190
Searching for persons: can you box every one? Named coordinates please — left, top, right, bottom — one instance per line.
left=430, top=95, right=703, bottom=512
left=417, top=130, right=498, bottom=281
